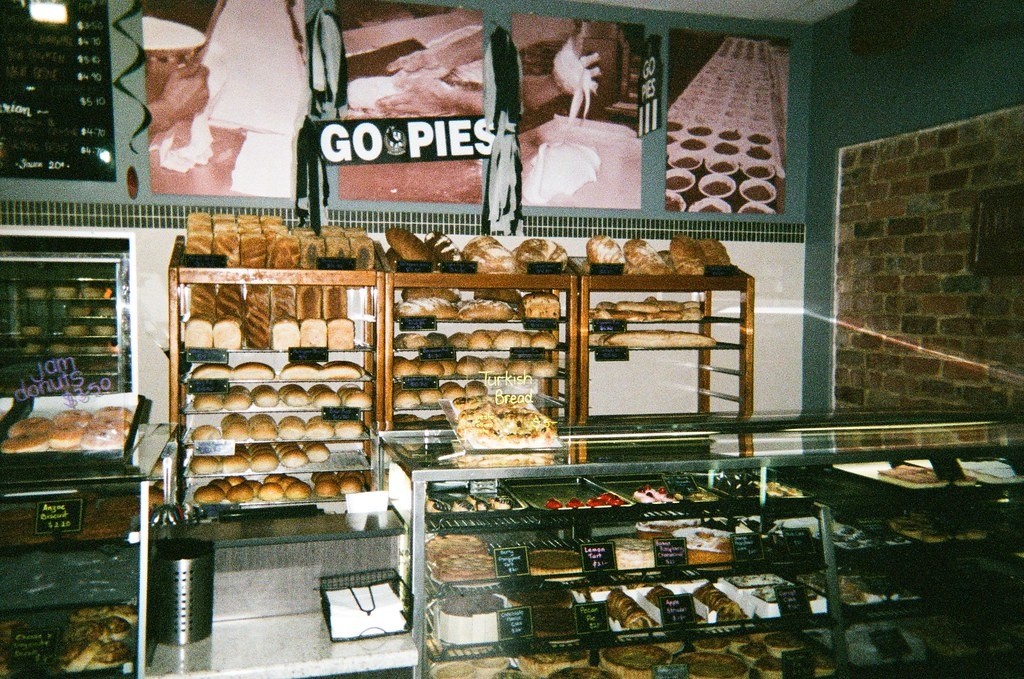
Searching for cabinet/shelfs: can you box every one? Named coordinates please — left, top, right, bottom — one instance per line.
left=165, top=234, right=386, bottom=523
left=381, top=414, right=1024, bottom=679
left=373, top=242, right=580, bottom=465
left=569, top=253, right=757, bottom=421
left=1, top=254, right=131, bottom=397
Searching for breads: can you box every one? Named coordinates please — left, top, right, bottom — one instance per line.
left=0, top=406, right=164, bottom=542
left=589, top=297, right=705, bottom=321
left=382, top=227, right=570, bottom=447
left=589, top=330, right=717, bottom=347
left=180, top=213, right=375, bottom=503
left=585, top=234, right=730, bottom=277
left=425, top=466, right=1024, bottom=679
left=20, top=286, right=115, bottom=353
left=0, top=603, right=137, bottom=679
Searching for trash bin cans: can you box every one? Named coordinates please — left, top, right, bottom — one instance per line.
left=148, top=538, right=215, bottom=645
